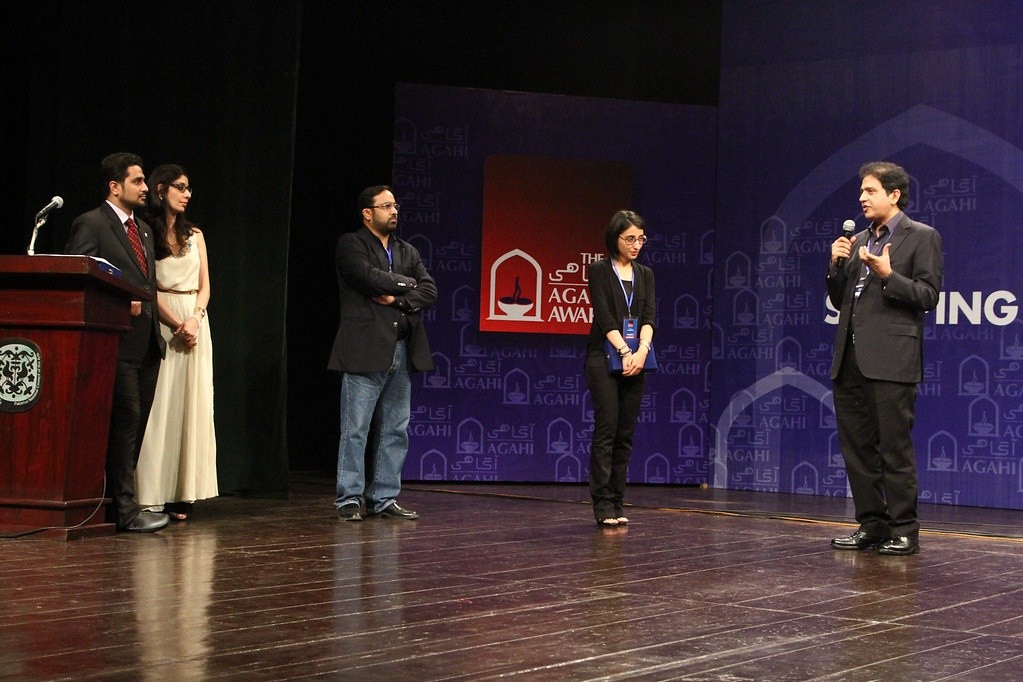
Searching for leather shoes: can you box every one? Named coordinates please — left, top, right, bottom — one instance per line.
left=830, top=531, right=890, bottom=550
left=338, top=504, right=363, bottom=520
left=878, top=536, right=920, bottom=554
left=170, top=511, right=187, bottom=520
left=367, top=502, right=419, bottom=519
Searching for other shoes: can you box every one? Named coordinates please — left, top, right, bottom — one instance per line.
left=126, top=512, right=170, bottom=532
left=597, top=516, right=629, bottom=526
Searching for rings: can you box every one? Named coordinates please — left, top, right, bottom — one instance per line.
left=181, top=331, right=184, bottom=333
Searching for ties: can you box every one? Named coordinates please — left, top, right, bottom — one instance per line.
left=125, top=218, right=148, bottom=279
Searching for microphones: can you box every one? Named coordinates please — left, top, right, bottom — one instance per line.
left=35, top=196, right=64, bottom=218
left=837, top=219, right=855, bottom=267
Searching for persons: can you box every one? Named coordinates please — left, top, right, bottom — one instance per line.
left=59, top=152, right=171, bottom=532
left=825, top=162, right=942, bottom=555
left=134, top=162, right=220, bottom=519
left=327, top=186, right=437, bottom=523
left=584, top=209, right=655, bottom=525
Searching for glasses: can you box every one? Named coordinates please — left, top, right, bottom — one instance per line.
left=170, top=183, right=192, bottom=194
left=368, top=202, right=400, bottom=211
left=618, top=235, right=647, bottom=245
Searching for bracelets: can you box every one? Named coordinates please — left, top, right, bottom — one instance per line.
left=194, top=306, right=205, bottom=317
left=639, top=338, right=651, bottom=351
left=188, top=315, right=201, bottom=329
left=620, top=349, right=632, bottom=356
left=616, top=342, right=627, bottom=353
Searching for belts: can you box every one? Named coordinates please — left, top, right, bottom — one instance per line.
left=397, top=335, right=405, bottom=341
